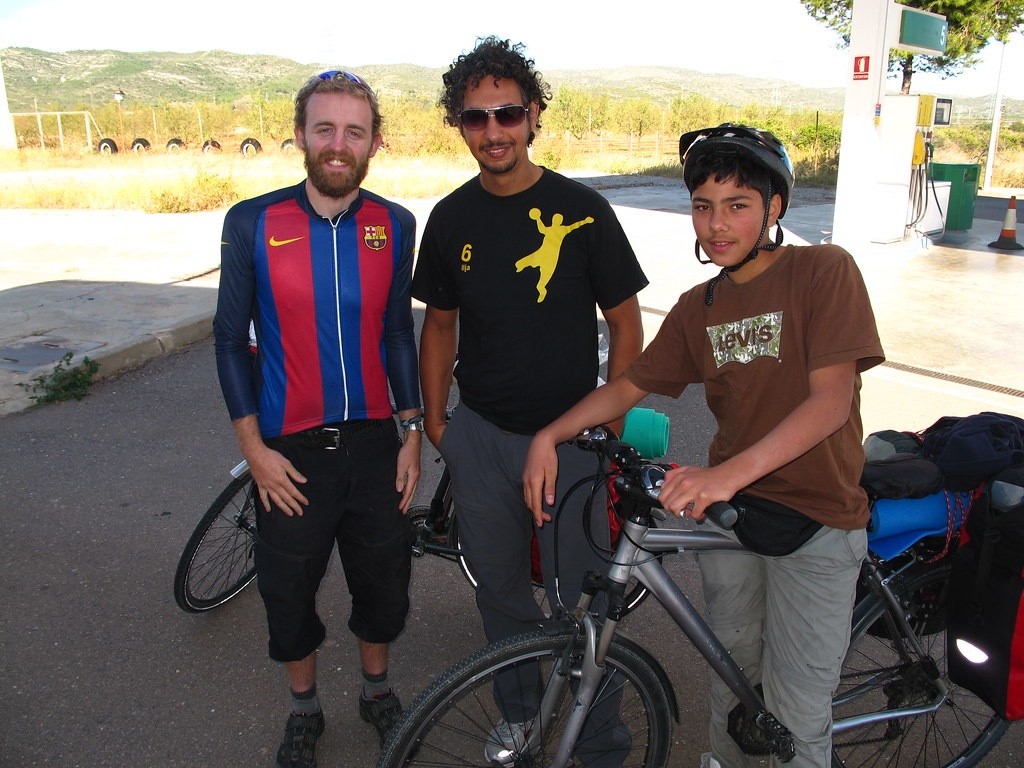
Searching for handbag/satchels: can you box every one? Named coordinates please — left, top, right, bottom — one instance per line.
left=726, top=492, right=823, bottom=557
left=851, top=411, right=1024, bottom=723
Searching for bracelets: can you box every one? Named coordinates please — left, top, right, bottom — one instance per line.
left=399, top=416, right=421, bottom=430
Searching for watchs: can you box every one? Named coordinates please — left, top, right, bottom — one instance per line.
left=402, top=422, right=424, bottom=432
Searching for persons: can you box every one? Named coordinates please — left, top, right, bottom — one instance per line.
left=521, top=122, right=887, bottom=768
left=212, top=70, right=423, bottom=768
left=412, top=35, right=649, bottom=768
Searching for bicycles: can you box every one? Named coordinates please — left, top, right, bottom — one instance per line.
left=371, top=425, right=1015, bottom=768
left=172, top=339, right=664, bottom=620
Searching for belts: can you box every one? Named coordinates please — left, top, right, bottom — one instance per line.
left=274, top=415, right=392, bottom=451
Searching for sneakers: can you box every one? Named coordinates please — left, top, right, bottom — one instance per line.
left=276, top=695, right=324, bottom=768
left=484, top=716, right=537, bottom=767
left=357, top=684, right=405, bottom=749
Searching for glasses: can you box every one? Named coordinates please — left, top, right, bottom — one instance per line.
left=457, top=104, right=530, bottom=131
left=308, top=70, right=371, bottom=91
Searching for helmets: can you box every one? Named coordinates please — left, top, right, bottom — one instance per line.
left=679, top=120, right=796, bottom=220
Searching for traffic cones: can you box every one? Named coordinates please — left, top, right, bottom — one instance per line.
left=987, top=197, right=1023, bottom=251
